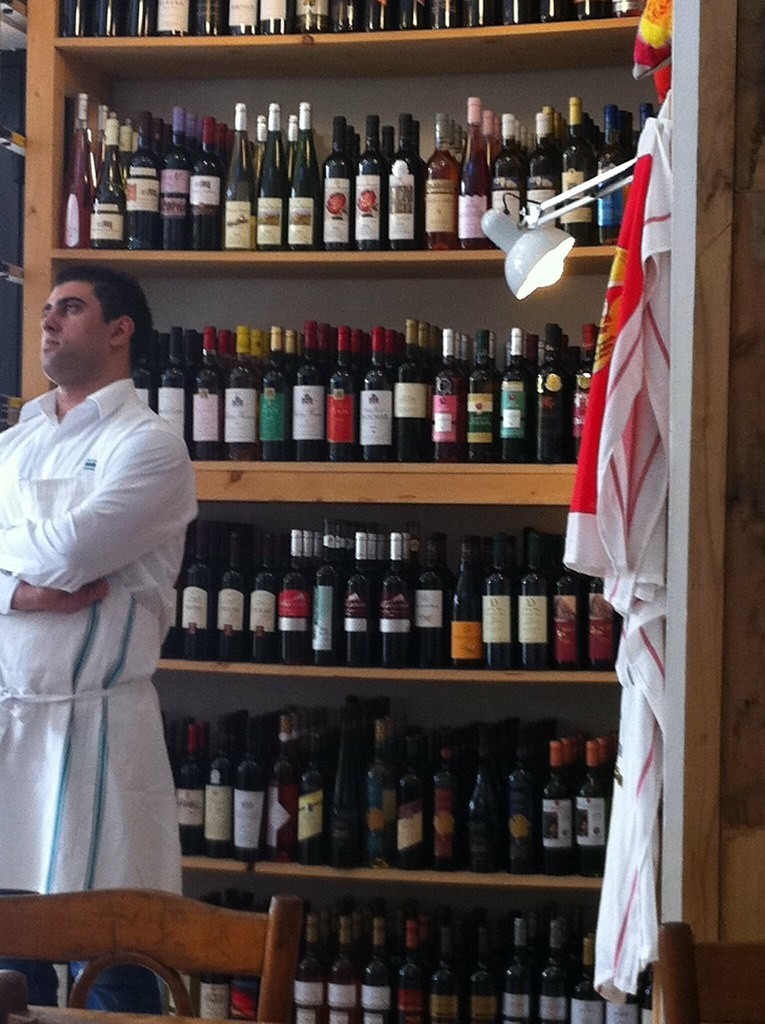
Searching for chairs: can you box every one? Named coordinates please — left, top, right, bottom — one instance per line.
left=0, top=889, right=303, bottom=1024
left=653, top=920, right=765, bottom=1024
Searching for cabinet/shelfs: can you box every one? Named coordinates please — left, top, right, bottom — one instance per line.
left=19, top=0, right=638, bottom=887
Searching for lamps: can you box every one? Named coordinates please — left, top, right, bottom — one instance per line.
left=482, top=156, right=636, bottom=298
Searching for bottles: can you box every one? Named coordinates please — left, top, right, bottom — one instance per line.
left=160, top=695, right=616, bottom=877
left=168, top=888, right=654, bottom=1024
left=61, top=0, right=648, bottom=37
left=63, top=92, right=655, bottom=253
left=157, top=520, right=624, bottom=671
left=129, top=319, right=598, bottom=463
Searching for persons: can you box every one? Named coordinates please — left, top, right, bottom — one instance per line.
left=0, top=264, right=199, bottom=1017
